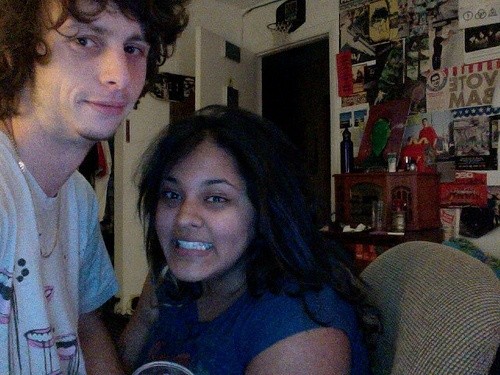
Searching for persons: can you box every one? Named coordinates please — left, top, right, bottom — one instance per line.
left=0, top=1, right=150, bottom=372
left=114, top=114, right=368, bottom=372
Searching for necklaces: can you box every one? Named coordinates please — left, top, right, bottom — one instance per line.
left=1, top=111, right=60, bottom=258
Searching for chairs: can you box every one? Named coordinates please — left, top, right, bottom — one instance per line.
left=349, top=239, right=500, bottom=375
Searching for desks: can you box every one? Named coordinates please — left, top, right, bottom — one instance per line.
left=319, top=227, right=445, bottom=279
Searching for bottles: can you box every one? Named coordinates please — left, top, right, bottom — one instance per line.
left=340, top=128, right=355, bottom=173
